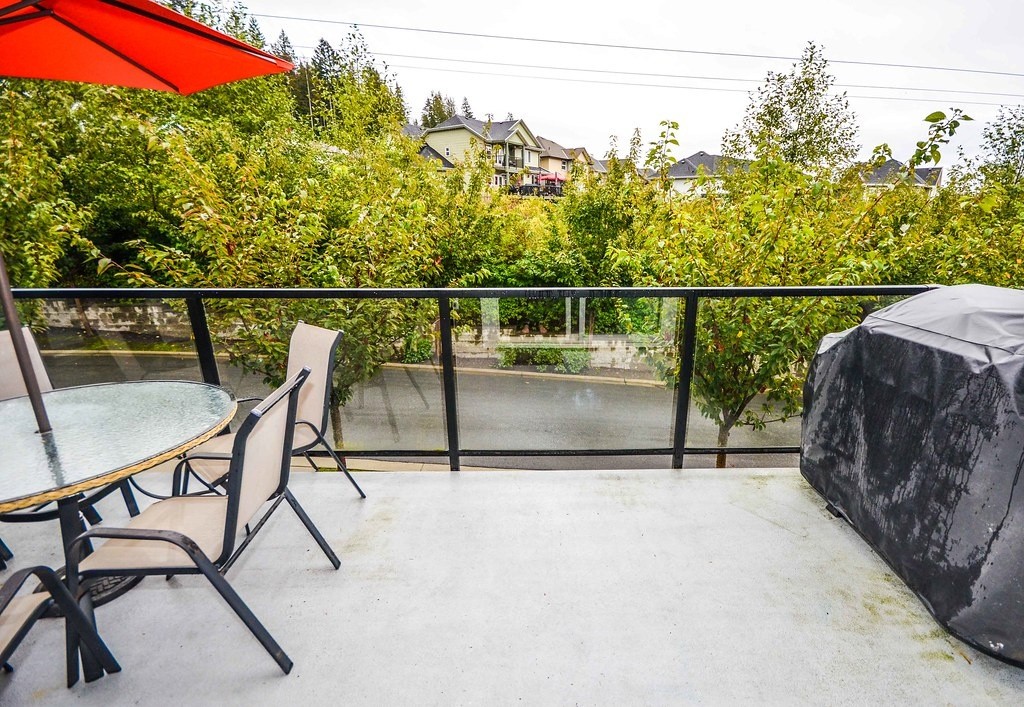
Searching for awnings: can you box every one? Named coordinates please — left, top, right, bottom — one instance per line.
left=539, top=172, right=565, bottom=182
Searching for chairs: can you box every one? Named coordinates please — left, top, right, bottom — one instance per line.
left=0, top=566, right=121, bottom=674
left=166, top=320, right=367, bottom=581
left=64, top=365, right=341, bottom=689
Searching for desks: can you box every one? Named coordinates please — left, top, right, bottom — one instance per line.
left=0, top=380, right=239, bottom=683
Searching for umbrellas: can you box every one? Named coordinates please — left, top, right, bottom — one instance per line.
left=0, top=0, right=296, bottom=564
